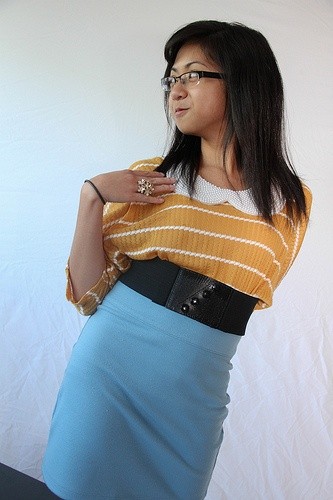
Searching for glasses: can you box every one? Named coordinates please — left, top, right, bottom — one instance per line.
left=160, top=70, right=225, bottom=92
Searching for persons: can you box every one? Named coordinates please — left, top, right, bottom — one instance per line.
left=45, top=17, right=315, bottom=499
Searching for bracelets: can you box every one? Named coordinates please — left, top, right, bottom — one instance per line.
left=83, top=177, right=109, bottom=207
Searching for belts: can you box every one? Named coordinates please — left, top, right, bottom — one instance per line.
left=123, top=257, right=255, bottom=336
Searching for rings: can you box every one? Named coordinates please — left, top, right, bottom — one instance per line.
left=135, top=177, right=156, bottom=198
